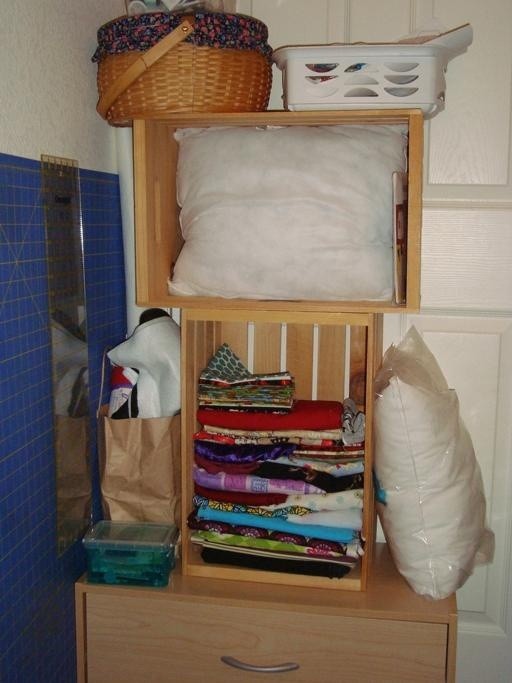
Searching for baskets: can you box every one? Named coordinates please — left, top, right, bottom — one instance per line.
left=272, top=41, right=452, bottom=120
left=94, top=8, right=274, bottom=128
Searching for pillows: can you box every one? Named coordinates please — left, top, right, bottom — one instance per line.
left=374, top=341, right=491, bottom=603
left=166, top=125, right=408, bottom=300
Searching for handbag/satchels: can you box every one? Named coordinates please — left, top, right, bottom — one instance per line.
left=94, top=343, right=185, bottom=529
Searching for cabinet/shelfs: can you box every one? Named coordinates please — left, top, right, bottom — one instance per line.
left=132, top=103, right=425, bottom=315
left=71, top=539, right=460, bottom=683
left=177, top=302, right=381, bottom=594
